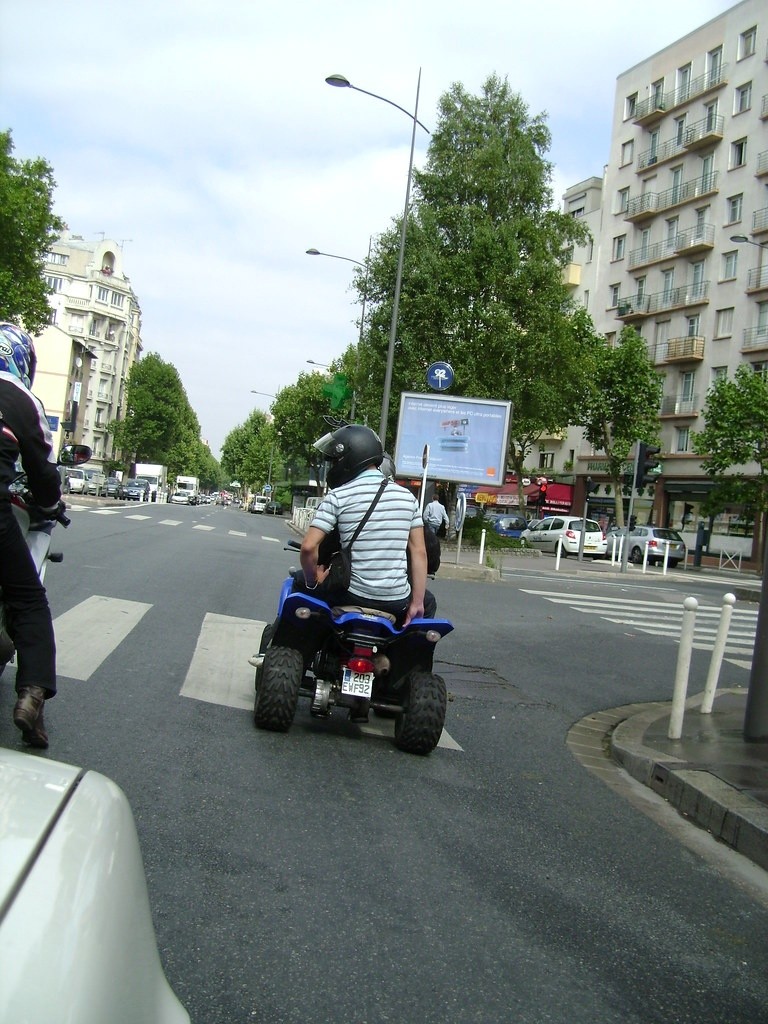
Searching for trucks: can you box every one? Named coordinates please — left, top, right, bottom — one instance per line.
left=176, top=475, right=200, bottom=506
left=134, top=463, right=167, bottom=502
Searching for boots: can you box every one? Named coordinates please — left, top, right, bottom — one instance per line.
left=13, top=685, right=48, bottom=748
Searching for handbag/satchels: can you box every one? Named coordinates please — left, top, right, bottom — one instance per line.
left=321, top=550, right=351, bottom=596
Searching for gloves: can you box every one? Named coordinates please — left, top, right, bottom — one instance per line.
left=34, top=498, right=66, bottom=521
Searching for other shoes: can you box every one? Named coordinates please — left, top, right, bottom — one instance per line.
left=249, top=656, right=264, bottom=668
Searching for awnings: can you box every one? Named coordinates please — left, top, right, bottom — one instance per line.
left=471, top=475, right=574, bottom=505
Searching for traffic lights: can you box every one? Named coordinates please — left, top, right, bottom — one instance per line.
left=539, top=484, right=548, bottom=505
left=634, top=439, right=662, bottom=484
left=628, top=515, right=637, bottom=531
left=588, top=481, right=601, bottom=492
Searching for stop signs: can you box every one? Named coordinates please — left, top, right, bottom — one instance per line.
left=423, top=444, right=428, bottom=469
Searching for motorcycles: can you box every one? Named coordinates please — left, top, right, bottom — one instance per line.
left=0, top=443, right=93, bottom=678
left=248, top=519, right=455, bottom=756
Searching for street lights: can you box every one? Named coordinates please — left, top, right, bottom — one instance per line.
left=325, top=66, right=430, bottom=455
left=306, top=234, right=372, bottom=425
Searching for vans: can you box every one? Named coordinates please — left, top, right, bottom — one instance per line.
left=118, top=478, right=150, bottom=502
left=249, top=495, right=271, bottom=514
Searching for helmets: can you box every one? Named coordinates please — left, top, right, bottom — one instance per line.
left=321, top=425, right=383, bottom=488
left=0, top=323, right=37, bottom=391
left=379, top=450, right=397, bottom=482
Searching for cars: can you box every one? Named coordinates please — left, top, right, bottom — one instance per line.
left=484, top=513, right=529, bottom=539
left=519, top=515, right=608, bottom=560
left=457, top=497, right=481, bottom=519
left=171, top=491, right=189, bottom=505
left=66, top=468, right=122, bottom=500
left=527, top=519, right=539, bottom=527
left=263, top=502, right=284, bottom=515
left=198, top=490, right=246, bottom=509
left=593, top=525, right=687, bottom=568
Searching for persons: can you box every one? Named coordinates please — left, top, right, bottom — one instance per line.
left=247, top=425, right=442, bottom=668
left=0, top=322, right=63, bottom=745
left=423, top=494, right=449, bottom=535
